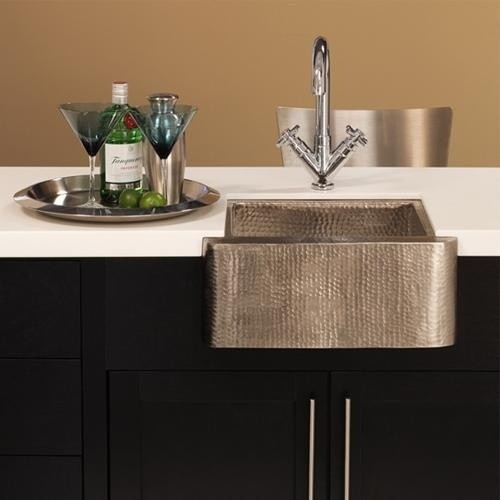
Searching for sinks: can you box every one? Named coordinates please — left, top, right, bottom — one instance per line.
left=202, top=199, right=457, bottom=348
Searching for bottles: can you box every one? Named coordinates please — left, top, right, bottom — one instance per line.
left=145, top=93, right=186, bottom=205
left=98, top=81, right=144, bottom=205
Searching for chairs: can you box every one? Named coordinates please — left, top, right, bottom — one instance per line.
left=275, top=107, right=453, bottom=168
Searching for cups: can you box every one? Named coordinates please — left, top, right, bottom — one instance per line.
left=130, top=105, right=197, bottom=206
left=59, top=102, right=129, bottom=208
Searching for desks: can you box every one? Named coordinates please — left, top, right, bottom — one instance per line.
left=2, top=163, right=500, bottom=260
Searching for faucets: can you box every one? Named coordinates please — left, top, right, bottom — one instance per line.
left=276, top=37, right=367, bottom=191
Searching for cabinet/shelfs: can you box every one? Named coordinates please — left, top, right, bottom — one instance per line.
left=106, top=371, right=500, bottom=500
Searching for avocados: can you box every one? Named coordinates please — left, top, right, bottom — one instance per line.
left=139, top=192, right=167, bottom=209
left=119, top=189, right=142, bottom=208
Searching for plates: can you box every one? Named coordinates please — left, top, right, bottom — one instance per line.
left=14, top=174, right=220, bottom=223
